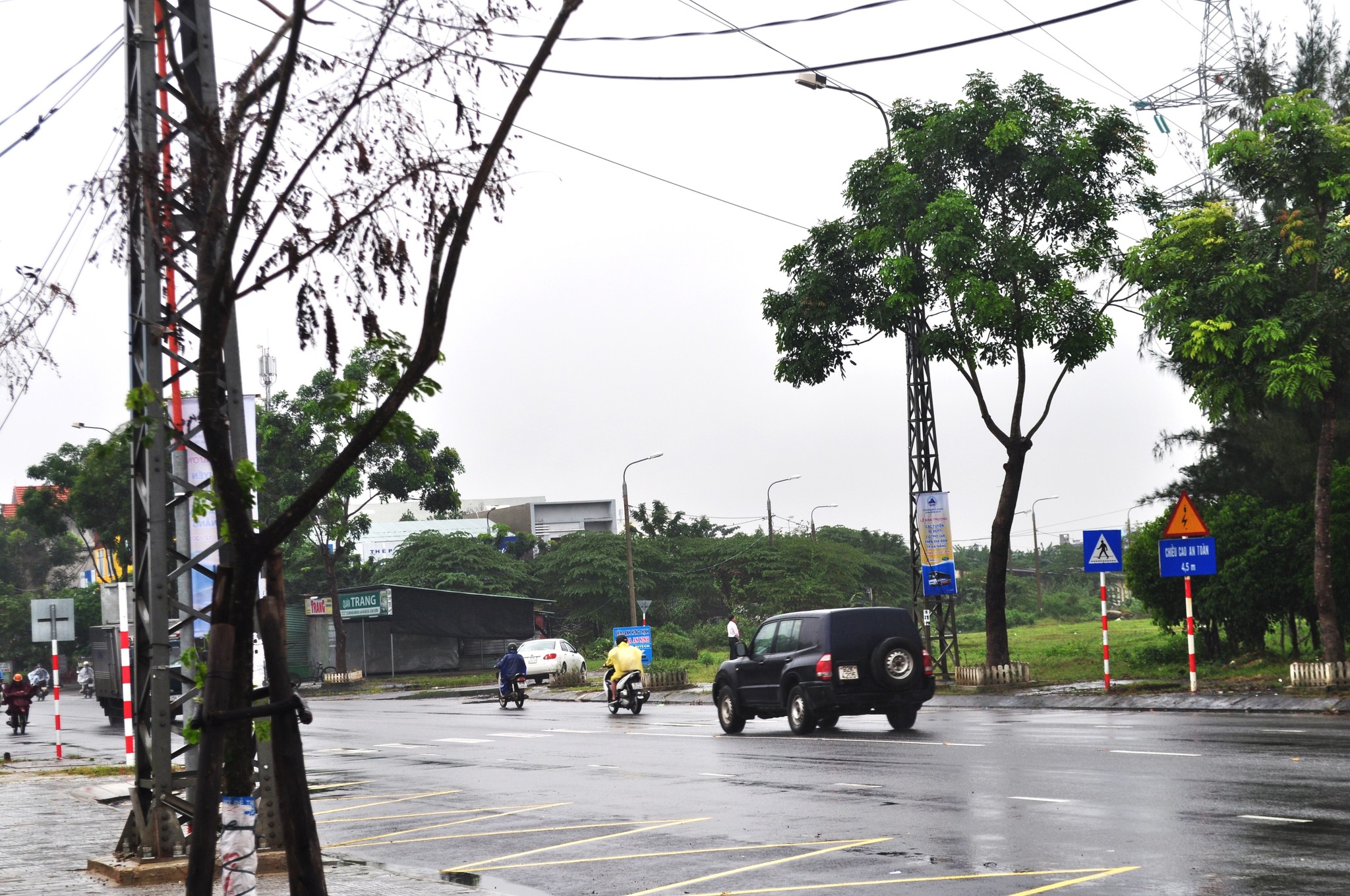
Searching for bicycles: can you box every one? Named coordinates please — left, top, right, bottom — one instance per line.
left=309, top=660, right=336, bottom=684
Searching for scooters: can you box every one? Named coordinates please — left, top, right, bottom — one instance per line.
left=5, top=687, right=31, bottom=734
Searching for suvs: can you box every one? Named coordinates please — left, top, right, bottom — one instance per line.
left=712, top=606, right=937, bottom=735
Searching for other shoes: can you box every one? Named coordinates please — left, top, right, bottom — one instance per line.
left=501, top=695, right=507, bottom=698
left=79, top=690, right=84, bottom=693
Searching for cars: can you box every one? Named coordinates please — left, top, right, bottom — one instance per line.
left=517, top=638, right=588, bottom=682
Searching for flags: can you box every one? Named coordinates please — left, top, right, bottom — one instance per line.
left=912, top=492, right=957, bottom=595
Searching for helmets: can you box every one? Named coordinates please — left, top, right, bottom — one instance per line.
left=616, top=634, right=628, bottom=643
left=13, top=674, right=23, bottom=682
left=36, top=663, right=42, bottom=667
left=84, top=661, right=89, bottom=665
left=508, top=643, right=517, bottom=650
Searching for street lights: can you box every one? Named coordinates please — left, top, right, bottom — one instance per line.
left=619, top=452, right=662, bottom=624
left=1032, top=495, right=1058, bottom=612
left=1005, top=508, right=1031, bottom=576
left=766, top=475, right=801, bottom=544
left=798, top=69, right=896, bottom=150
left=811, top=503, right=837, bottom=535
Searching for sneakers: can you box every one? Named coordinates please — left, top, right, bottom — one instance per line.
left=607, top=698, right=618, bottom=704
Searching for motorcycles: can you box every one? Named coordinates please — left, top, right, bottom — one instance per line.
left=33, top=673, right=49, bottom=701
left=600, top=664, right=651, bottom=713
left=76, top=670, right=96, bottom=699
left=492, top=657, right=528, bottom=707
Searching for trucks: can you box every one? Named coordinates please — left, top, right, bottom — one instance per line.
left=92, top=619, right=192, bottom=721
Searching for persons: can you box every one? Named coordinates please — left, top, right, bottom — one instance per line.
left=496, top=643, right=527, bottom=697
left=0, top=670, right=4, bottom=702
left=727, top=614, right=740, bottom=660
left=602, top=634, right=644, bottom=701
left=27, top=663, right=50, bottom=687
left=77, top=661, right=94, bottom=693
left=3, top=673, right=37, bottom=724
